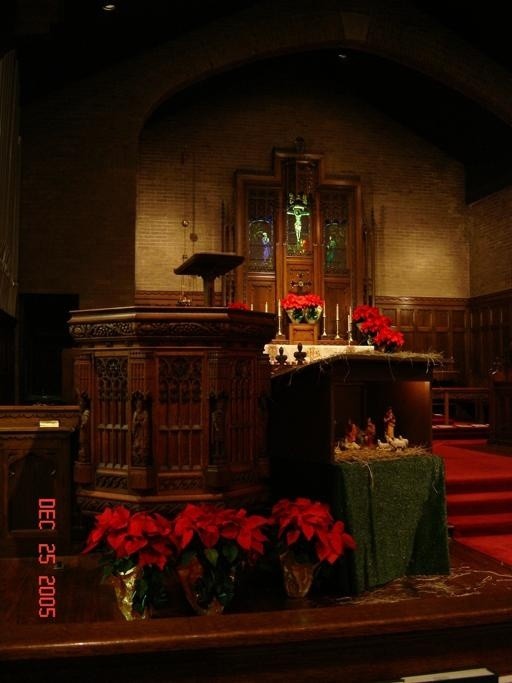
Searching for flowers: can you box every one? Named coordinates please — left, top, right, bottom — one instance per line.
left=281, top=293, right=323, bottom=320
left=80, top=496, right=356, bottom=614
left=351, top=304, right=405, bottom=352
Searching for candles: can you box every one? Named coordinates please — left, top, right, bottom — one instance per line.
left=251, top=299, right=281, bottom=316
left=323, top=299, right=351, bottom=331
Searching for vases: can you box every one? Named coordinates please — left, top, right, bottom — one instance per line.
left=101, top=546, right=325, bottom=620
left=284, top=306, right=322, bottom=324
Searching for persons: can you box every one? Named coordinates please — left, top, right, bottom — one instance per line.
left=262, top=233, right=269, bottom=263
left=212, top=401, right=226, bottom=457
left=132, top=401, right=149, bottom=466
left=367, top=418, right=375, bottom=445
left=326, top=236, right=336, bottom=267
left=78, top=396, right=91, bottom=461
left=287, top=209, right=310, bottom=242
left=344, top=418, right=356, bottom=443
left=383, top=407, right=397, bottom=438
